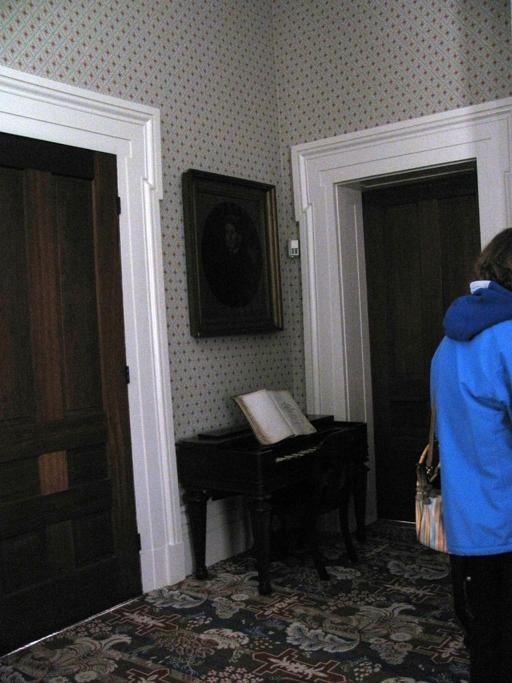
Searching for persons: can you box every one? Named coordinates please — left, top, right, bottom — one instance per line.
left=212, top=216, right=257, bottom=306
left=427, top=225, right=512, bottom=683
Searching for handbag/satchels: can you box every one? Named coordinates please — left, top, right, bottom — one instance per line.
left=415, top=441, right=446, bottom=552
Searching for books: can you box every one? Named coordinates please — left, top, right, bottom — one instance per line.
left=231, top=388, right=318, bottom=446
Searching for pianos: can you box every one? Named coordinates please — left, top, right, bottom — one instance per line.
left=175, top=413, right=369, bottom=595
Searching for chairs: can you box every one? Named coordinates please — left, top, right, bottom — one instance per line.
left=269, top=426, right=355, bottom=582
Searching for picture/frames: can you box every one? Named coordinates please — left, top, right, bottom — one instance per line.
left=181, top=169, right=284, bottom=339
